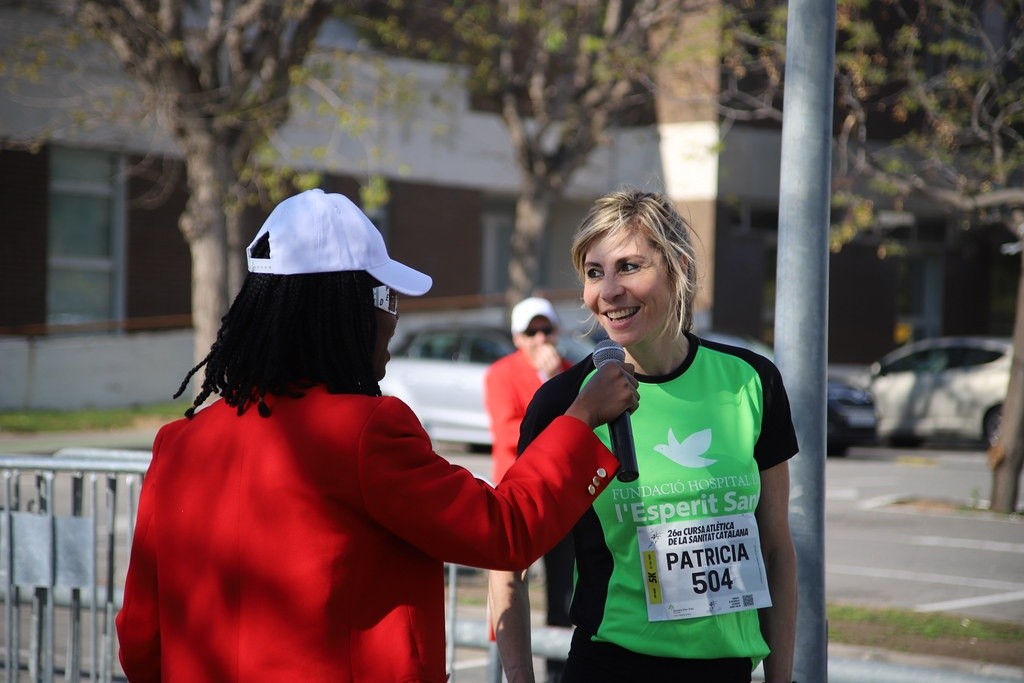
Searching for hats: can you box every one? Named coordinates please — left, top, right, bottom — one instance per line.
left=511, top=296, right=558, bottom=335
left=246, top=188, right=432, bottom=297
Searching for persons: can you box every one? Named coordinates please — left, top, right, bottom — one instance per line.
left=481, top=186, right=799, bottom=683
left=115, top=187, right=641, bottom=683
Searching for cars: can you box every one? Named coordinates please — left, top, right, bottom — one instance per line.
left=871, top=338, right=1014, bottom=448
left=378, top=302, right=883, bottom=456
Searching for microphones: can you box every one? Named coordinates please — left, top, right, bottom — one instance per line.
left=592, top=339, right=639, bottom=482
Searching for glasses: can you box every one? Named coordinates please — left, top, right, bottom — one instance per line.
left=522, top=324, right=554, bottom=338
left=362, top=285, right=399, bottom=316
